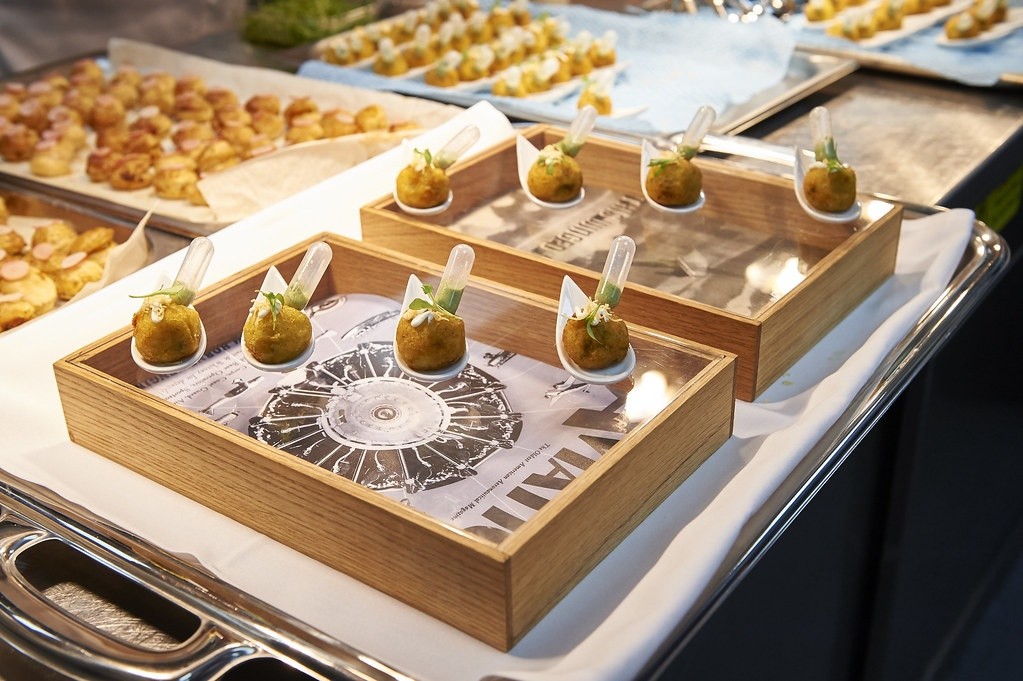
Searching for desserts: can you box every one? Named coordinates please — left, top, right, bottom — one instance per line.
left=0, top=0, right=1005, bottom=373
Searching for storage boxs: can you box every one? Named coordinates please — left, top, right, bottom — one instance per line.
left=360, top=122, right=910, bottom=402
left=52, top=229, right=740, bottom=651
